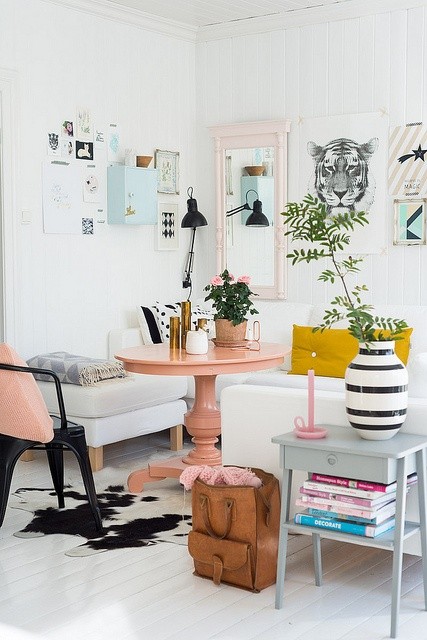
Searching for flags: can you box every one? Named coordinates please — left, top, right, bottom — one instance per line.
left=212, top=318, right=248, bottom=345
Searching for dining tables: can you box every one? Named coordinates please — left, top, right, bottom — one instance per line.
left=281, top=195, right=408, bottom=442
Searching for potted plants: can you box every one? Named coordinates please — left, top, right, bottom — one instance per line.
left=155, top=149, right=179, bottom=194
left=388, top=123, right=427, bottom=196
left=393, top=200, right=426, bottom=246
left=157, top=202, right=180, bottom=252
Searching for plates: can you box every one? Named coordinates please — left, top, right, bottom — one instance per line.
left=273, top=423, right=427, bottom=640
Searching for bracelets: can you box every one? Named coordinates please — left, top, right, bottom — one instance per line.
left=136, top=155, right=153, bottom=167
left=246, top=166, right=266, bottom=176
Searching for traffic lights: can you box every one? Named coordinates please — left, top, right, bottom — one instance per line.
left=291, top=327, right=412, bottom=380
left=25, top=351, right=129, bottom=387
left=141, top=299, right=217, bottom=348
left=0, top=343, right=55, bottom=444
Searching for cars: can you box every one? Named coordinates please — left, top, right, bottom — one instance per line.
left=185, top=331, right=208, bottom=355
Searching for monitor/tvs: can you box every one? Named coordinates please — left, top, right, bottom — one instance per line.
left=294, top=473, right=419, bottom=539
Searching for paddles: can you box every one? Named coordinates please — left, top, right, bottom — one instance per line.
left=4, top=450, right=325, bottom=562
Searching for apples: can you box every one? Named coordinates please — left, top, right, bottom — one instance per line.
left=307, top=367, right=314, bottom=432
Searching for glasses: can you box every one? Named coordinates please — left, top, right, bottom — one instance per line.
left=181, top=187, right=208, bottom=302
left=224, top=190, right=269, bottom=227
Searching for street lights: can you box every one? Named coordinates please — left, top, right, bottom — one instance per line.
left=188, top=464, right=281, bottom=593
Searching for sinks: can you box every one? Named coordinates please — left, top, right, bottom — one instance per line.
left=19, top=369, right=187, bottom=472
left=136, top=312, right=348, bottom=439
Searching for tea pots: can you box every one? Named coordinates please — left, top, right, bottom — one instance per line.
left=210, top=120, right=289, bottom=303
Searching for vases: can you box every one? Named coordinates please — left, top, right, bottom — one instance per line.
left=203, top=269, right=259, bottom=324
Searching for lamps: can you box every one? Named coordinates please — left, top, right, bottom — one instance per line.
left=0, top=345, right=105, bottom=537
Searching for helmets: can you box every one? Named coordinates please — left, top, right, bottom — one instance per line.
left=211, top=339, right=250, bottom=347
